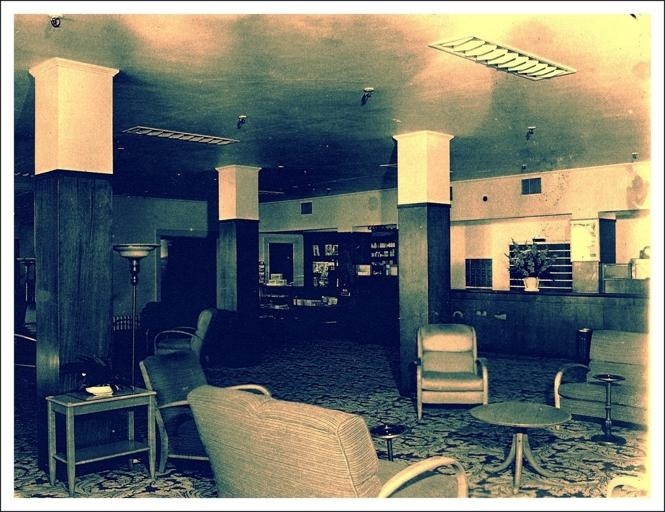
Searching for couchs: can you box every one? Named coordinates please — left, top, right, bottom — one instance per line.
left=553, top=329, right=647, bottom=435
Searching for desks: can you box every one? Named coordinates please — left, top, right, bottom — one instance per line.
left=45, top=387, right=157, bottom=498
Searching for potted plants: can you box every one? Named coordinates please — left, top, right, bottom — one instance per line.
left=505, top=239, right=558, bottom=292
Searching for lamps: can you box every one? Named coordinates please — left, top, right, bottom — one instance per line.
left=113, top=243, right=162, bottom=465
left=16, top=257, right=37, bottom=320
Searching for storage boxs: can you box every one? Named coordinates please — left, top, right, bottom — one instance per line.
left=631, top=258, right=651, bottom=281
left=601, top=262, right=632, bottom=281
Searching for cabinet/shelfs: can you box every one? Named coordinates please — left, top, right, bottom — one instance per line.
left=306, top=231, right=398, bottom=290
left=258, top=284, right=353, bottom=325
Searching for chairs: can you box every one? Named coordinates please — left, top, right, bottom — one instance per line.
left=415, top=323, right=488, bottom=421
left=139, top=350, right=273, bottom=473
left=187, top=383, right=470, bottom=498
left=139, top=305, right=218, bottom=362
